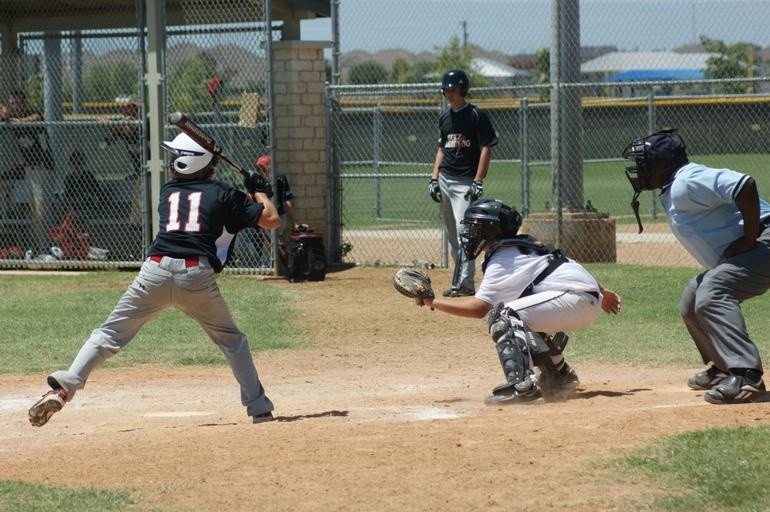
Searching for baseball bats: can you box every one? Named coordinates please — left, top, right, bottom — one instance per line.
left=169, top=111, right=252, bottom=178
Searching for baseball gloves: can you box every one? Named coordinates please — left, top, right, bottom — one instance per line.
left=393, top=268, right=435, bottom=306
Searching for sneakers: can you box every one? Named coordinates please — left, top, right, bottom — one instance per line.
left=704, top=374, right=765, bottom=404
left=29, top=388, right=68, bottom=427
left=687, top=365, right=728, bottom=389
left=252, top=412, right=274, bottom=423
left=484, top=367, right=579, bottom=405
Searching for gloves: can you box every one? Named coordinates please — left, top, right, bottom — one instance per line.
left=243, top=171, right=274, bottom=202
left=464, top=180, right=483, bottom=202
left=428, top=179, right=442, bottom=203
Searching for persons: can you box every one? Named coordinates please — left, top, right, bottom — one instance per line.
left=418, top=198, right=623, bottom=406
left=110, top=102, right=155, bottom=177
left=429, top=71, right=498, bottom=296
left=29, top=127, right=281, bottom=428
left=1, top=89, right=57, bottom=177
left=63, top=151, right=102, bottom=213
left=247, top=155, right=294, bottom=268
left=623, top=127, right=770, bottom=406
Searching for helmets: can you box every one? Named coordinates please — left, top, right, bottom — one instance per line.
left=459, top=199, right=522, bottom=261
left=441, top=69, right=470, bottom=97
left=161, top=133, right=221, bottom=180
left=623, top=128, right=689, bottom=191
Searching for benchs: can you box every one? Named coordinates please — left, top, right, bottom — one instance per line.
left=0, top=175, right=144, bottom=258
